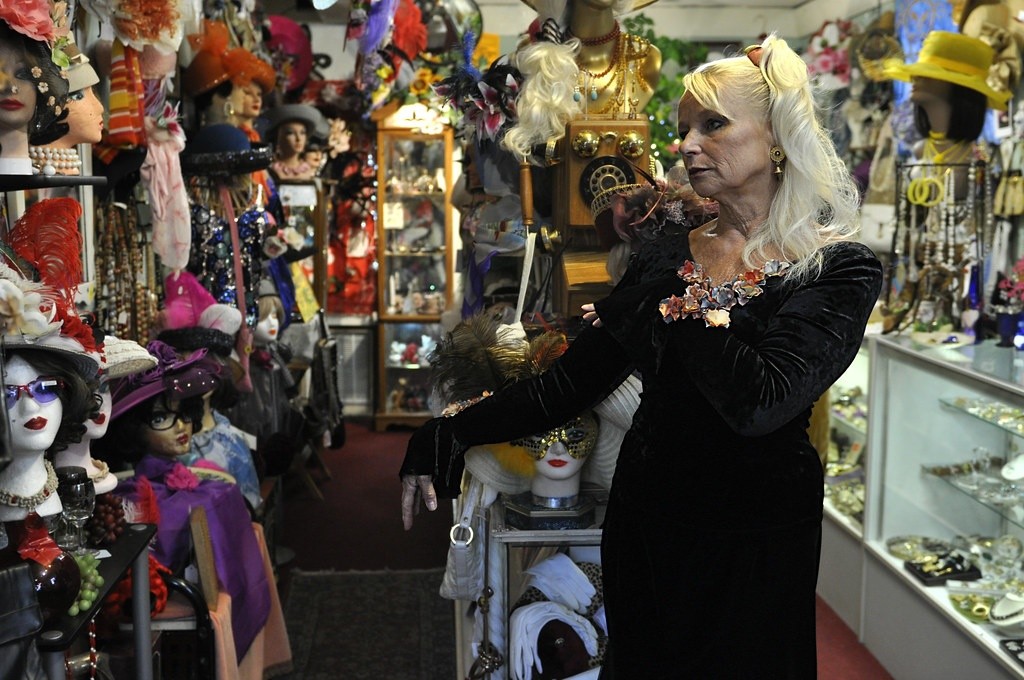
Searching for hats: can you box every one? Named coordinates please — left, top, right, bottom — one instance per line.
left=424, top=313, right=644, bottom=496
left=898, top=30, right=1015, bottom=102
left=0, top=0, right=330, bottom=420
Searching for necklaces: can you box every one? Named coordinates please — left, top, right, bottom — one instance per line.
left=0, top=459, right=59, bottom=532
left=28, top=147, right=83, bottom=178
left=274, top=158, right=309, bottom=175
left=990, top=590, right=1024, bottom=620
left=561, top=19, right=626, bottom=115
left=94, top=189, right=166, bottom=349
left=90, top=458, right=109, bottom=482
left=878, top=130, right=993, bottom=336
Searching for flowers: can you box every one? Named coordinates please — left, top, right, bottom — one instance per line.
left=0, top=0, right=69, bottom=67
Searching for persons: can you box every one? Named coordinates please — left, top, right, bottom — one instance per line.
left=400, top=30, right=883, bottom=680
left=0, top=263, right=260, bottom=521
left=858, top=29, right=1014, bottom=200
left=0, top=0, right=104, bottom=174
left=429, top=313, right=644, bottom=507
left=258, top=105, right=331, bottom=179
left=498, top=0, right=663, bottom=174
left=181, top=19, right=276, bottom=153
left=254, top=280, right=285, bottom=346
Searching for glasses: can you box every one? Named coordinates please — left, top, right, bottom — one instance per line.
left=3, top=372, right=66, bottom=412
left=135, top=403, right=204, bottom=431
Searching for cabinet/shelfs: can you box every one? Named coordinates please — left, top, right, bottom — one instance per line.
left=817, top=318, right=1024, bottom=680
left=0, top=0, right=455, bottom=680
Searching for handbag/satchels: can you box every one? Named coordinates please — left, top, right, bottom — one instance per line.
left=438, top=479, right=486, bottom=603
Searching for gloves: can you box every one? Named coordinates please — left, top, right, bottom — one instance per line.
left=505, top=555, right=599, bottom=680
